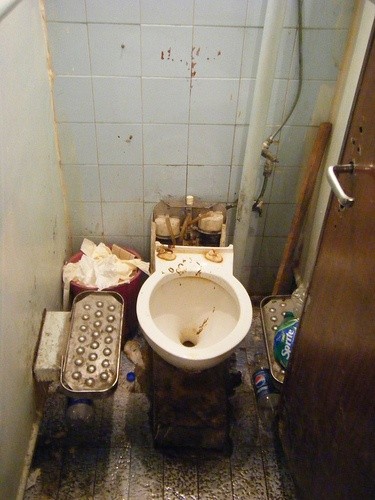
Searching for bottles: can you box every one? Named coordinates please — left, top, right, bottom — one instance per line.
left=250, top=351, right=283, bottom=421
left=273, top=312, right=300, bottom=369
left=66, top=399, right=95, bottom=430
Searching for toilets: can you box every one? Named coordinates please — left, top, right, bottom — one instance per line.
left=136, top=197, right=253, bottom=370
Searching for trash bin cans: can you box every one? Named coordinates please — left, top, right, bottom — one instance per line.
left=66, top=246, right=143, bottom=337
